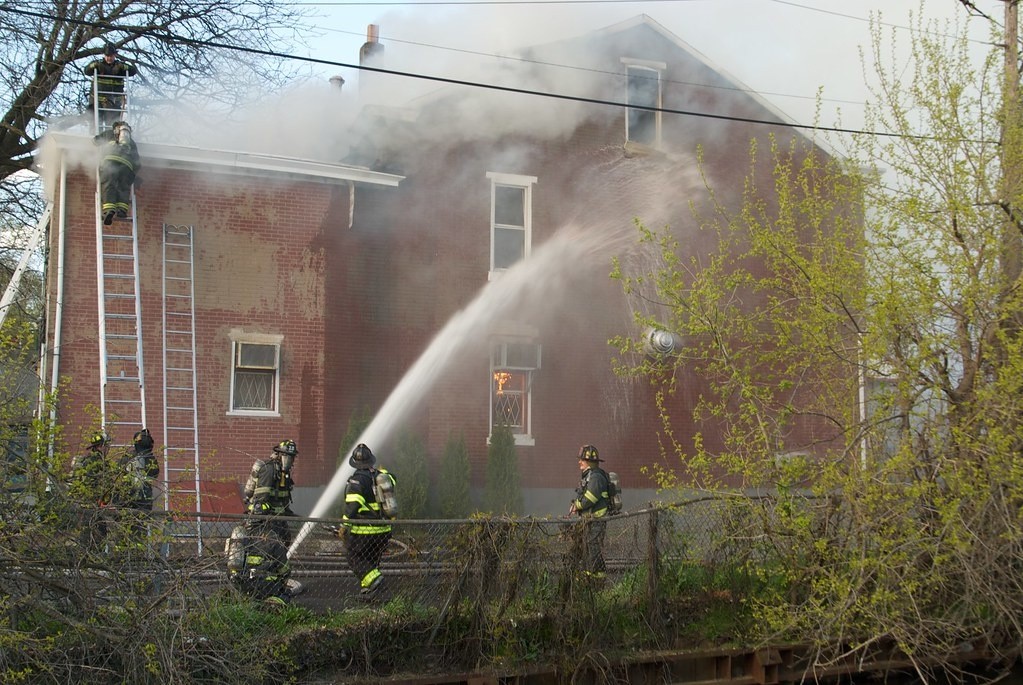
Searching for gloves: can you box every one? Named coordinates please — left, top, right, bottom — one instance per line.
left=115, top=64, right=124, bottom=73
left=339, top=526, right=349, bottom=542
left=95, top=62, right=104, bottom=73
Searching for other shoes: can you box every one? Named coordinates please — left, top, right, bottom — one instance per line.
left=104, top=212, right=114, bottom=225
left=118, top=209, right=126, bottom=217
left=368, top=579, right=389, bottom=602
left=354, top=594, right=370, bottom=602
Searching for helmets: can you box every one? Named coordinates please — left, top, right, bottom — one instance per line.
left=577, top=445, right=605, bottom=463
left=349, top=444, right=376, bottom=469
left=134, top=429, right=154, bottom=454
left=274, top=440, right=299, bottom=456
left=86, top=431, right=111, bottom=449
left=104, top=42, right=117, bottom=55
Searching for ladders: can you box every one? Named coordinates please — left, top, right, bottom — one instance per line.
left=160, top=222, right=205, bottom=564
left=90, top=63, right=147, bottom=454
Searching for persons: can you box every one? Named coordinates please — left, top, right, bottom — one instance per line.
left=339, top=443, right=396, bottom=602
left=74, top=432, right=112, bottom=552
left=94, top=121, right=141, bottom=225
left=554, top=445, right=611, bottom=589
left=221, top=440, right=298, bottom=605
left=84, top=41, right=136, bottom=134
left=114, top=428, right=160, bottom=536
